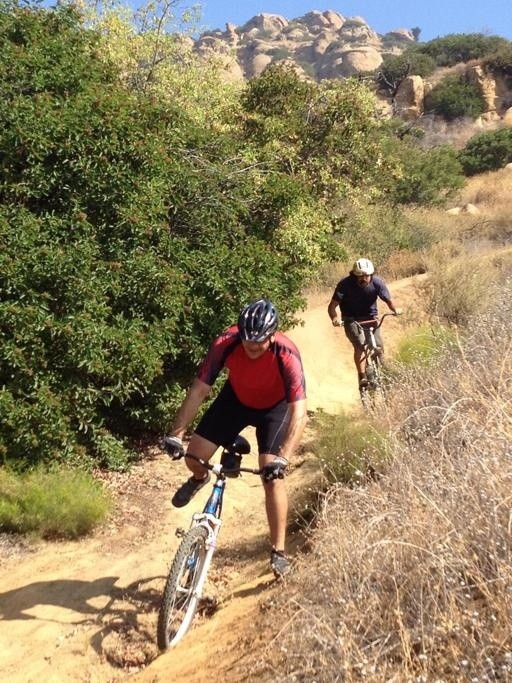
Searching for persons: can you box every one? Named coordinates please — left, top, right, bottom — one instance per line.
left=159, top=298, right=309, bottom=582
left=327, top=258, right=404, bottom=393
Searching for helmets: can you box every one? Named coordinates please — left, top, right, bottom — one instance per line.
left=353, top=257, right=375, bottom=276
left=237, top=297, right=280, bottom=342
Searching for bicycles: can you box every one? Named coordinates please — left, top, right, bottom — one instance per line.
left=156, top=432, right=288, bottom=650
left=337, top=312, right=398, bottom=413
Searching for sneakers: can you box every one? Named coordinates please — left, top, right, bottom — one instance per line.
left=359, top=378, right=369, bottom=386
left=171, top=470, right=211, bottom=506
left=270, top=550, right=290, bottom=575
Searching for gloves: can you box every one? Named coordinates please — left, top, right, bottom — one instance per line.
left=158, top=435, right=185, bottom=460
left=262, top=455, right=289, bottom=481
